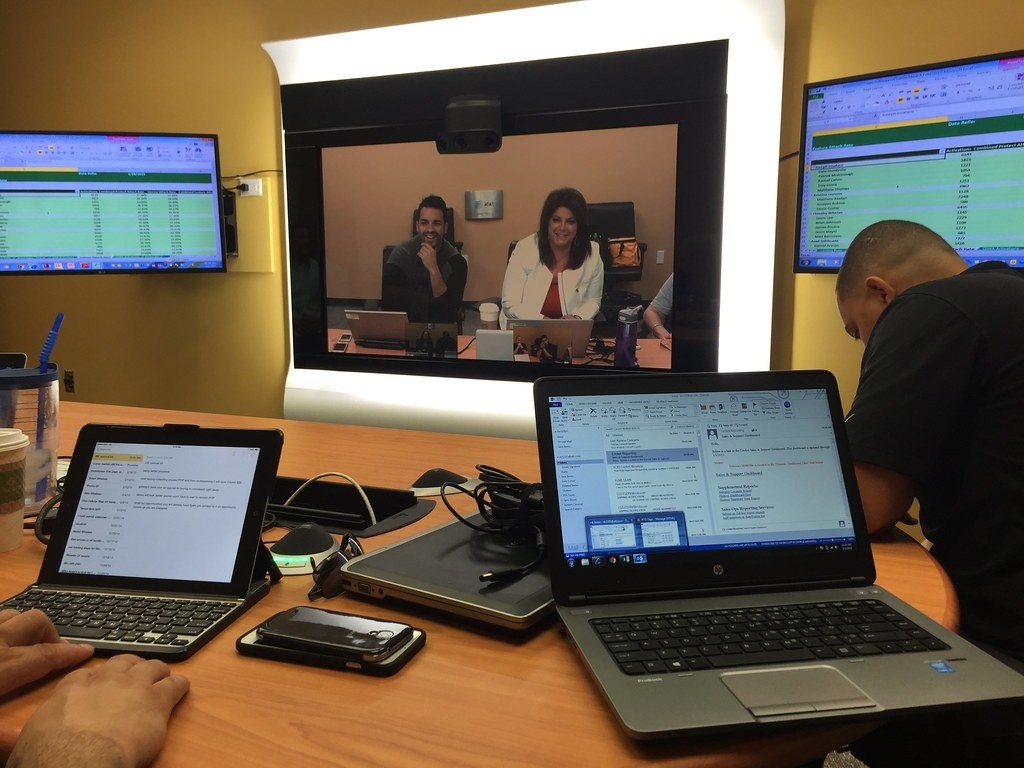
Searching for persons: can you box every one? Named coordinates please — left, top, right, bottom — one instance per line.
left=415, top=330, right=434, bottom=352
left=513, top=335, right=528, bottom=354
left=380, top=195, right=468, bottom=323
left=835, top=219, right=1024, bottom=768
left=435, top=331, right=457, bottom=353
left=0, top=608, right=190, bottom=768
left=642, top=272, right=673, bottom=339
left=499, top=186, right=604, bottom=330
left=536, top=335, right=557, bottom=361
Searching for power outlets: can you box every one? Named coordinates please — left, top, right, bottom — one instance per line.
left=238, top=178, right=263, bottom=197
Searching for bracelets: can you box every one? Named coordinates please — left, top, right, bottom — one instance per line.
left=652, top=324, right=664, bottom=335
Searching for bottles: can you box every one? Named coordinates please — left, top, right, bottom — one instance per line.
left=615, top=304, right=642, bottom=367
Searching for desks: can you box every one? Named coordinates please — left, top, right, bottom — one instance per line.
left=327, top=329, right=672, bottom=370
left=0, top=399, right=963, bottom=768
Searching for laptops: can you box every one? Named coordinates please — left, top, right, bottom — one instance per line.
left=506, top=319, right=594, bottom=359
left=535, top=368, right=1024, bottom=740
left=344, top=309, right=409, bottom=348
left=0, top=423, right=283, bottom=660
left=341, top=507, right=556, bottom=631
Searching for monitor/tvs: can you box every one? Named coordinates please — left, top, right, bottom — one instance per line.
left=793, top=48, right=1024, bottom=274
left=0, top=130, right=227, bottom=276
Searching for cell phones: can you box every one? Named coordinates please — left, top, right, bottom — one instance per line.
left=332, top=342, right=348, bottom=353
left=236, top=606, right=427, bottom=679
left=338, top=333, right=352, bottom=342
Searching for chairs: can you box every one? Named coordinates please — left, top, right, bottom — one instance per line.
left=582, top=202, right=649, bottom=291
left=381, top=241, right=465, bottom=335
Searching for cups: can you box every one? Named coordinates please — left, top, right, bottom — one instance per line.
left=0, top=428, right=30, bottom=552
left=0, top=361, right=60, bottom=511
left=479, top=304, right=499, bottom=329
